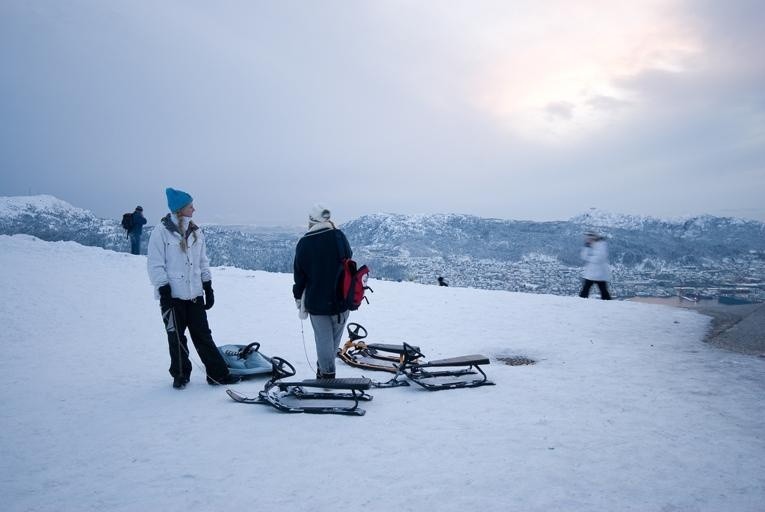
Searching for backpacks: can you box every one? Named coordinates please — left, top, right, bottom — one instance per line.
left=333, top=229, right=374, bottom=312
left=122, top=213, right=135, bottom=240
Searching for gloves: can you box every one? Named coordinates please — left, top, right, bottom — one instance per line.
left=159, top=284, right=173, bottom=311
left=203, top=280, right=215, bottom=310
left=295, top=299, right=309, bottom=319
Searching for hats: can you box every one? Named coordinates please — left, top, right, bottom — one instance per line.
left=135, top=206, right=142, bottom=211
left=307, top=205, right=331, bottom=225
left=584, top=231, right=595, bottom=239
left=166, top=187, right=193, bottom=214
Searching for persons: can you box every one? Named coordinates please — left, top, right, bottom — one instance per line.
left=146, top=187, right=243, bottom=389
left=292, top=204, right=353, bottom=379
left=128, top=205, right=147, bottom=255
left=579, top=230, right=612, bottom=300
left=437, top=277, right=448, bottom=286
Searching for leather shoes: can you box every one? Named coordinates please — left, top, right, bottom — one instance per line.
left=172, top=377, right=190, bottom=390
left=206, top=374, right=241, bottom=385
